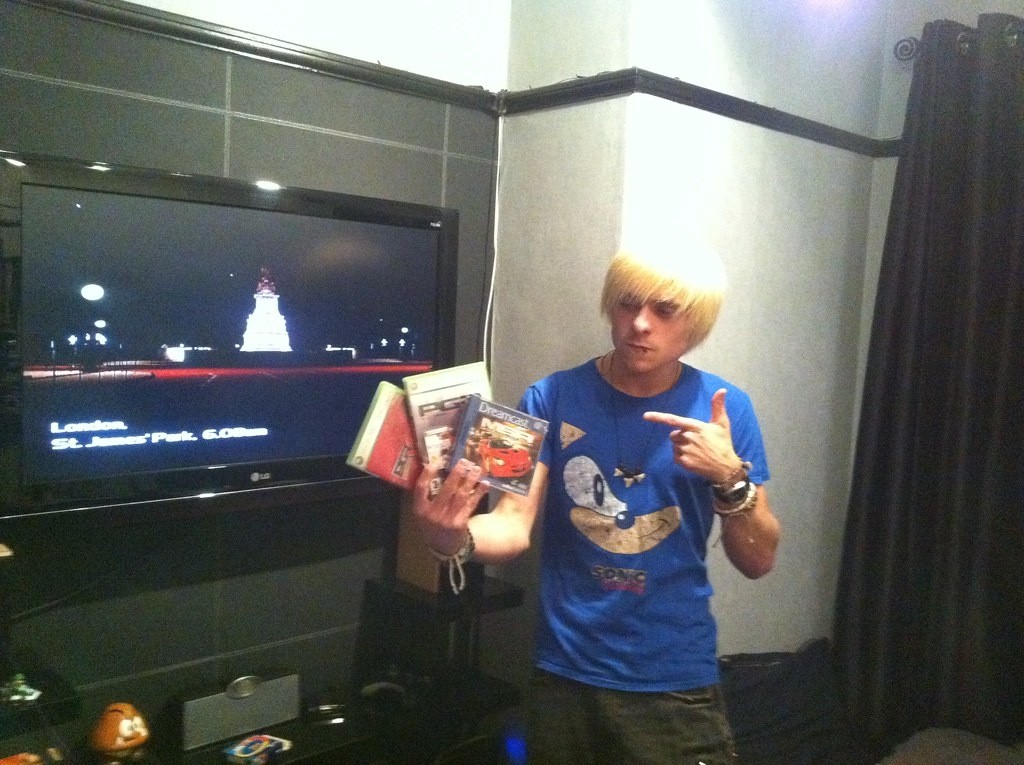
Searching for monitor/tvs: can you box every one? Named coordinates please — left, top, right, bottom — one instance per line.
left=0, top=149, right=459, bottom=538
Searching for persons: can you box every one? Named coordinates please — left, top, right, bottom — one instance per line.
left=413, top=233, right=779, bottom=765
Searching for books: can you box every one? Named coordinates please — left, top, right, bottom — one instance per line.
left=346, top=362, right=502, bottom=496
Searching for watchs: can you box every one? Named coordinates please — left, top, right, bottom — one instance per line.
left=712, top=476, right=750, bottom=504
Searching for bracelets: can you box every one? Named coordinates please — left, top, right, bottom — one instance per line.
left=713, top=461, right=753, bottom=493
left=426, top=528, right=475, bottom=596
left=711, top=483, right=757, bottom=548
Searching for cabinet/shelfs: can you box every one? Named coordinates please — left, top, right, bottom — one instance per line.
left=0, top=566, right=524, bottom=765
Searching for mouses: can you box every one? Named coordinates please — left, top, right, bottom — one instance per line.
left=359, top=681, right=405, bottom=700
